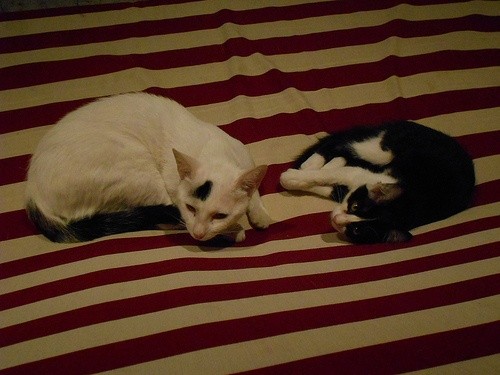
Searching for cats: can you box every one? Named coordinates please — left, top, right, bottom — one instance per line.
left=279, top=120, right=475, bottom=244
left=23, top=91, right=272, bottom=243
